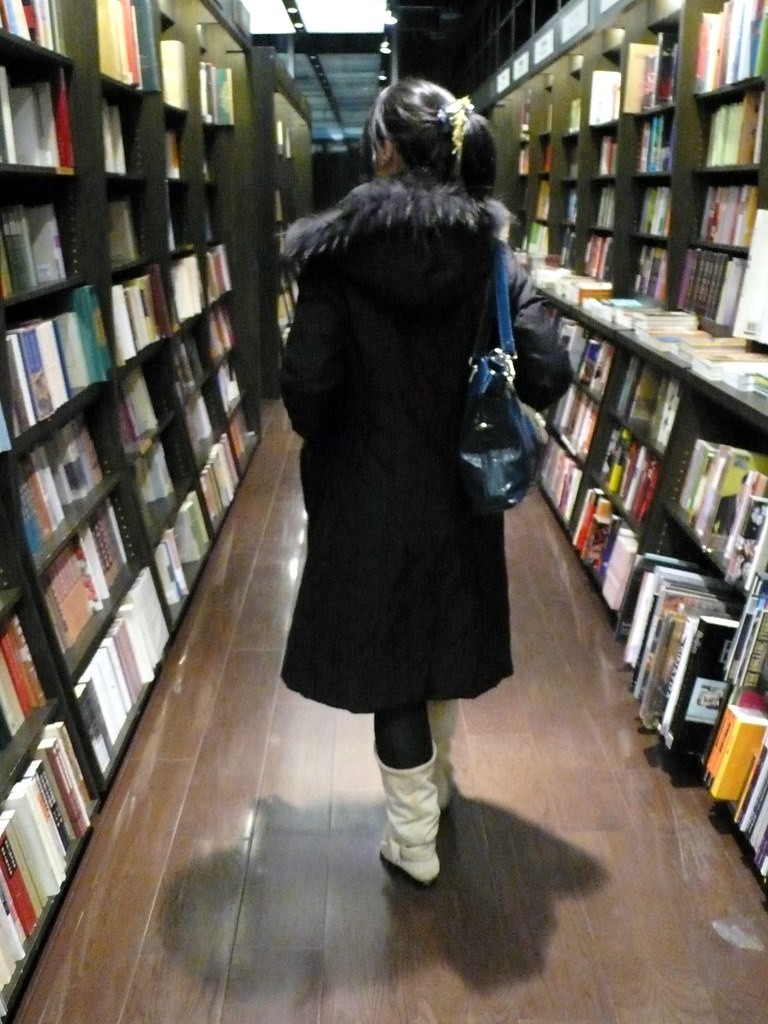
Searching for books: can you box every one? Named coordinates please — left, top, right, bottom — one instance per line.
left=488, top=0, right=768, bottom=883
left=0, top=0, right=295, bottom=1017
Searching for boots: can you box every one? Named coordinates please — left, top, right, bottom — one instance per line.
left=373, top=699, right=461, bottom=886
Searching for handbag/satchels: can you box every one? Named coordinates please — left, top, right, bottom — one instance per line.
left=454, top=238, right=551, bottom=518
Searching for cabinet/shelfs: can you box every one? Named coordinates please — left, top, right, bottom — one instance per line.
left=0, top=0, right=314, bottom=1024
left=469, top=0, right=768, bottom=910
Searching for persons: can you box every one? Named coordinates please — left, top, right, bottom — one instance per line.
left=277, top=78, right=571, bottom=886
